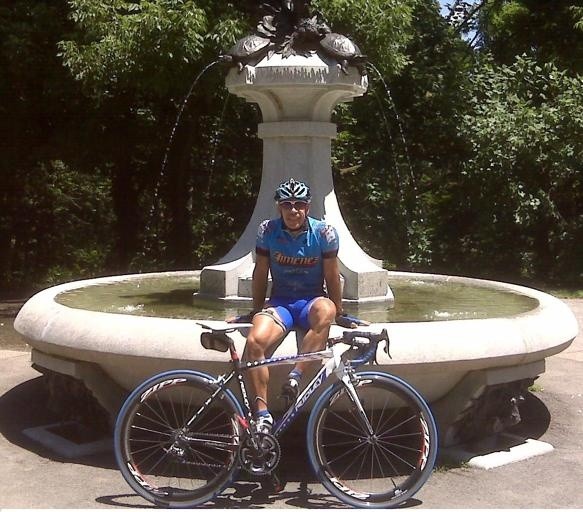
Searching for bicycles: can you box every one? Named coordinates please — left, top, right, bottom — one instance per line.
left=113, top=322, right=439, bottom=509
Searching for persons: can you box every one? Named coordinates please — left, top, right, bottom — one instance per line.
left=227, top=178, right=370, bottom=437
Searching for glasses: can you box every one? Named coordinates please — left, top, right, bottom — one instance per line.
left=281, top=202, right=307, bottom=210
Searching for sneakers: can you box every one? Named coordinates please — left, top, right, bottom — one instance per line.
left=282, top=374, right=300, bottom=398
left=251, top=413, right=274, bottom=434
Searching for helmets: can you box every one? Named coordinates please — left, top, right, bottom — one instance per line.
left=274, top=177, right=311, bottom=203
